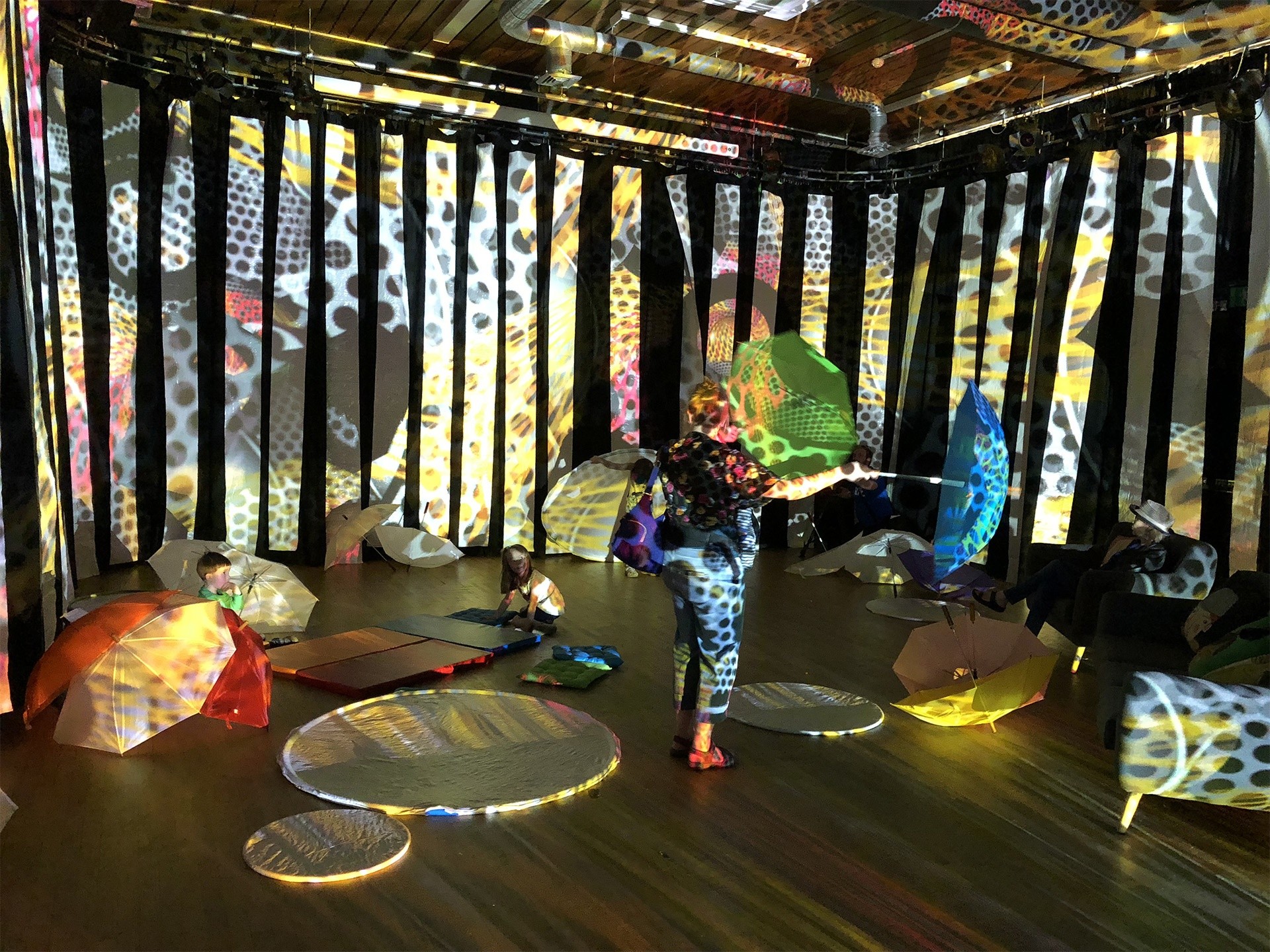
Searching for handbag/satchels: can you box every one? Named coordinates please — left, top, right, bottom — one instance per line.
left=611, top=494, right=669, bottom=576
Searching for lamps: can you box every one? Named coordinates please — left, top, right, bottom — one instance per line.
left=285, top=72, right=322, bottom=110
left=1071, top=110, right=1110, bottom=139
left=199, top=59, right=237, bottom=103
left=1221, top=69, right=1267, bottom=115
left=1009, top=123, right=1043, bottom=156
left=760, top=150, right=782, bottom=174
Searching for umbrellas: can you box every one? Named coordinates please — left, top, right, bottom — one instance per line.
left=783, top=513, right=1004, bottom=601
left=539, top=448, right=671, bottom=559
left=732, top=328, right=857, bottom=480
left=879, top=380, right=1010, bottom=578
left=375, top=502, right=466, bottom=573
left=324, top=505, right=404, bottom=571
left=326, top=499, right=361, bottom=547
left=23, top=538, right=320, bottom=758
left=891, top=604, right=1057, bottom=735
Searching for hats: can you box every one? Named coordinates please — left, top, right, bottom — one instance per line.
left=1129, top=500, right=1175, bottom=535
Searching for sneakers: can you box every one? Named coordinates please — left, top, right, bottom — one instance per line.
left=624, top=563, right=638, bottom=578
left=259, top=634, right=266, bottom=641
left=640, top=571, right=659, bottom=576
left=270, top=636, right=299, bottom=646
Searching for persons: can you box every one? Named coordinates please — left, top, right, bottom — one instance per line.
left=972, top=500, right=1172, bottom=636
left=76, top=521, right=133, bottom=579
left=483, top=544, right=565, bottom=635
left=591, top=456, right=666, bottom=577
left=837, top=446, right=894, bottom=542
left=196, top=554, right=244, bottom=618
left=659, top=378, right=881, bottom=769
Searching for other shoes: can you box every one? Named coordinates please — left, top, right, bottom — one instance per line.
left=532, top=629, right=547, bottom=636
left=515, top=627, right=523, bottom=632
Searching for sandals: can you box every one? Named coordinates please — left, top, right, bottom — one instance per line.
left=688, top=742, right=736, bottom=770
left=670, top=736, right=694, bottom=760
left=971, top=588, right=1006, bottom=613
